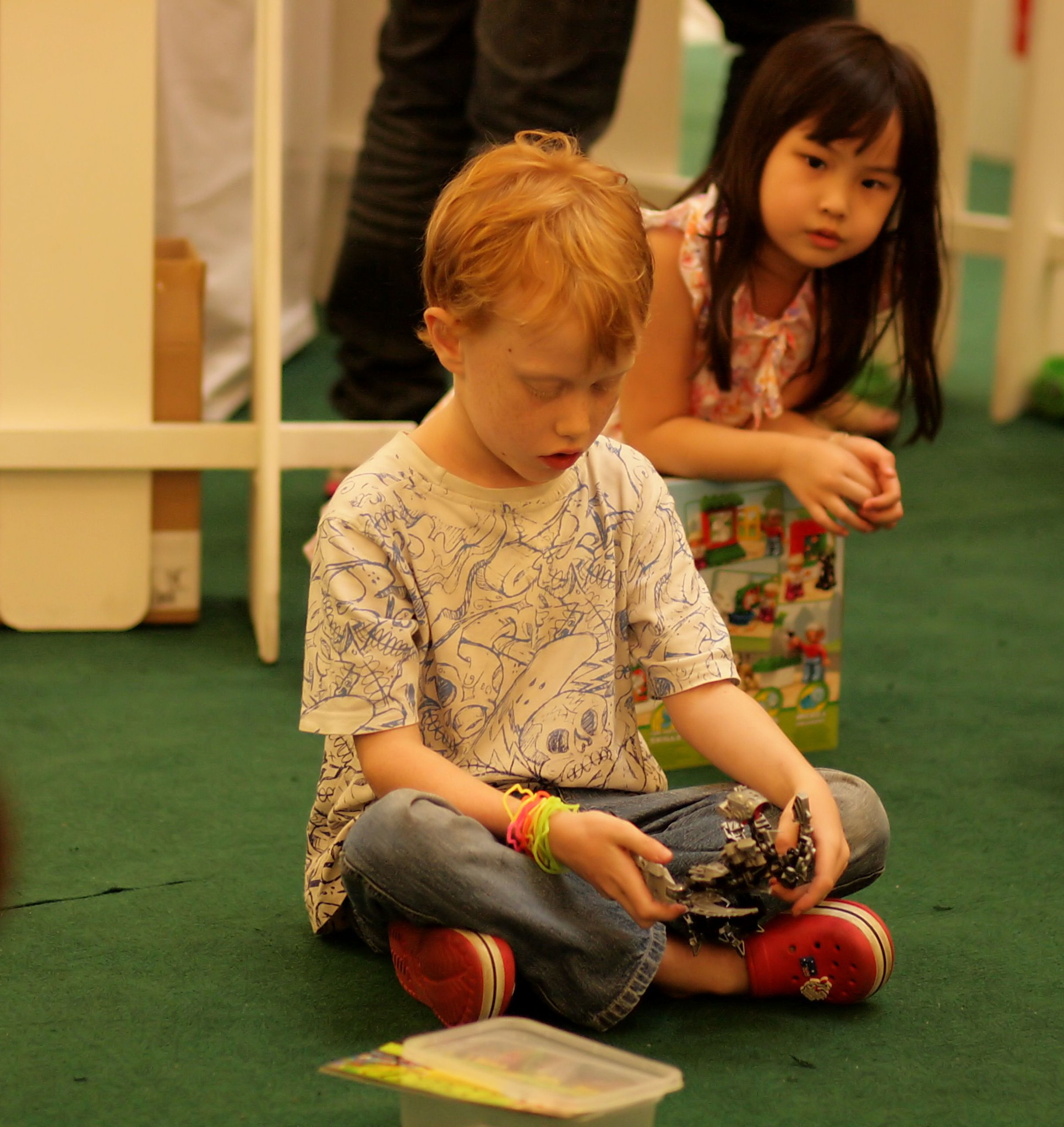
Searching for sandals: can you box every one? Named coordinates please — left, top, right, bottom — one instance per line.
left=386, top=907, right=518, bottom=1030
left=729, top=894, right=893, bottom=1012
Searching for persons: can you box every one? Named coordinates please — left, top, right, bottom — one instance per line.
left=323, top=0, right=637, bottom=423
left=602, top=21, right=946, bottom=535
left=708, top=0, right=863, bottom=191
left=298, top=127, right=895, bottom=1028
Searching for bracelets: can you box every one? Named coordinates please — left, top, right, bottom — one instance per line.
left=830, top=429, right=848, bottom=447
left=507, top=774, right=569, bottom=875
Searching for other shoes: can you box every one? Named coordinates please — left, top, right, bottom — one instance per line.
left=821, top=389, right=903, bottom=456
